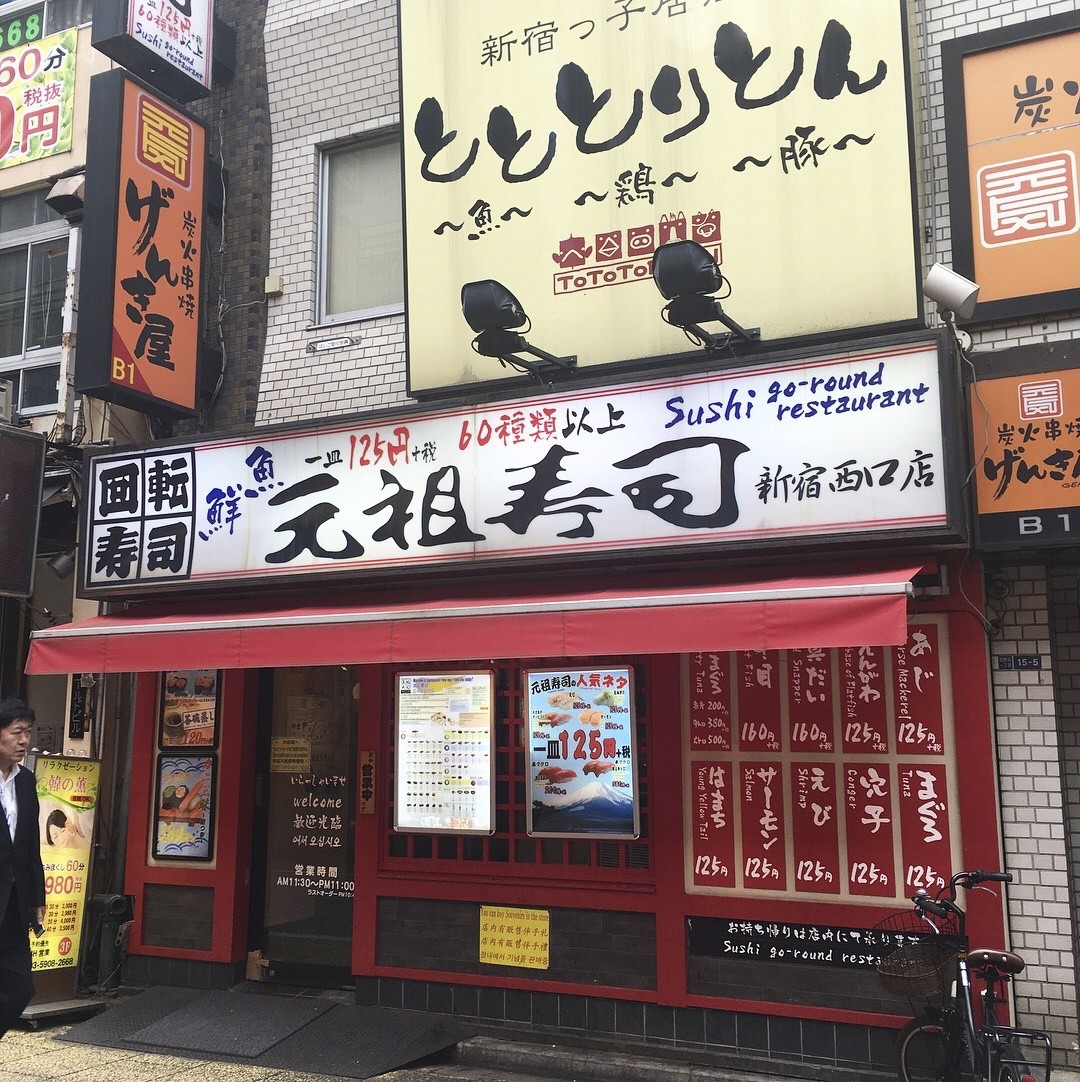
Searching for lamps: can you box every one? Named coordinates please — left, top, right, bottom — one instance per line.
left=460, top=279, right=577, bottom=374
left=652, top=239, right=759, bottom=350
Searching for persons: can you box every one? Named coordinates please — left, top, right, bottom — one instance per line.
left=46, top=809, right=88, bottom=847
left=0, top=697, right=47, bottom=1039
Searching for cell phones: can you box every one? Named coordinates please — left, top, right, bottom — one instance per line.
left=29, top=912, right=45, bottom=939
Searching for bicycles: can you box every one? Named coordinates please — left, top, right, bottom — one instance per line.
left=871, top=868, right=1051, bottom=1082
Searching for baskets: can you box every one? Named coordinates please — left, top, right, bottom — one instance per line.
left=864, top=909, right=967, bottom=1000
